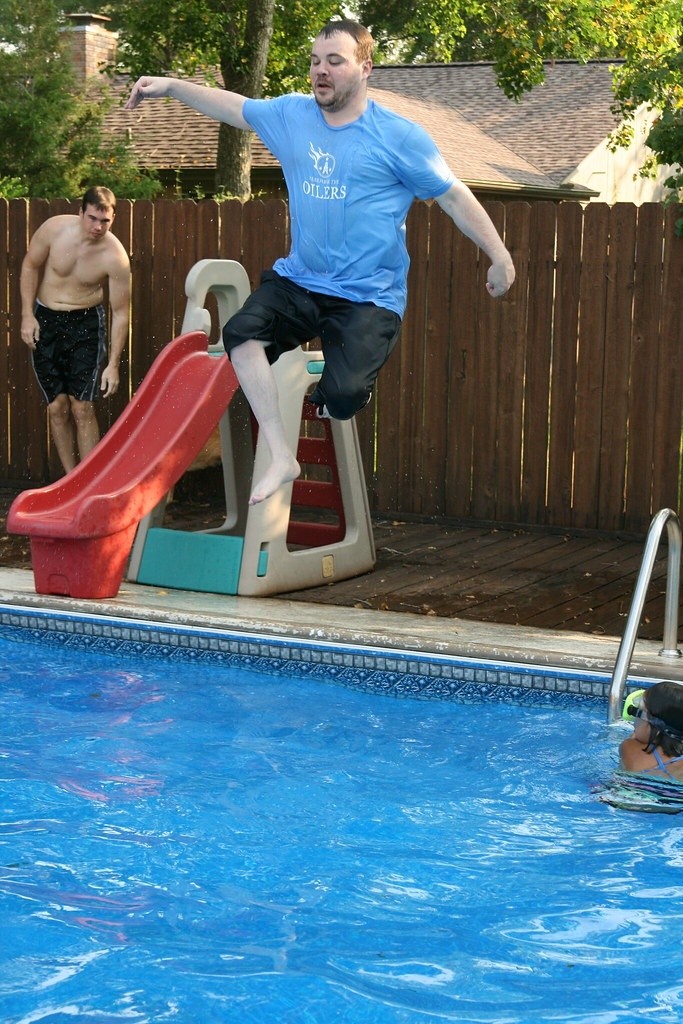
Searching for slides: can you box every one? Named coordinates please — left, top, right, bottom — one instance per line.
left=6, top=330, right=240, bottom=541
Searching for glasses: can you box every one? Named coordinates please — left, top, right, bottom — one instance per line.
left=622, top=689, right=648, bottom=721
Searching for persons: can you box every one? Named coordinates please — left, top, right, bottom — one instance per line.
left=619, top=682, right=683, bottom=780
left=125, top=20, right=516, bottom=504
left=20, top=187, right=132, bottom=473
